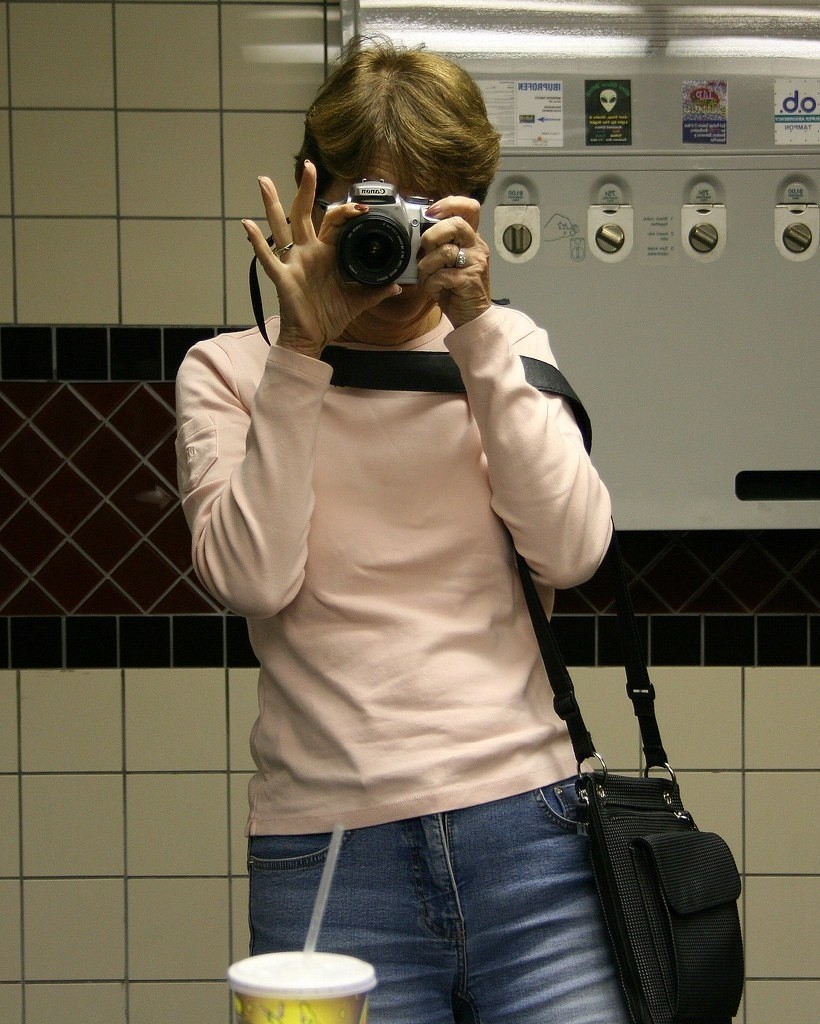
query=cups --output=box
[228,951,376,1024]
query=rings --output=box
[272,243,293,257]
[455,246,466,269]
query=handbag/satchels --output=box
[572,771,744,1024]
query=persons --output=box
[172,34,633,1024]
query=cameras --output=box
[333,178,451,286]
